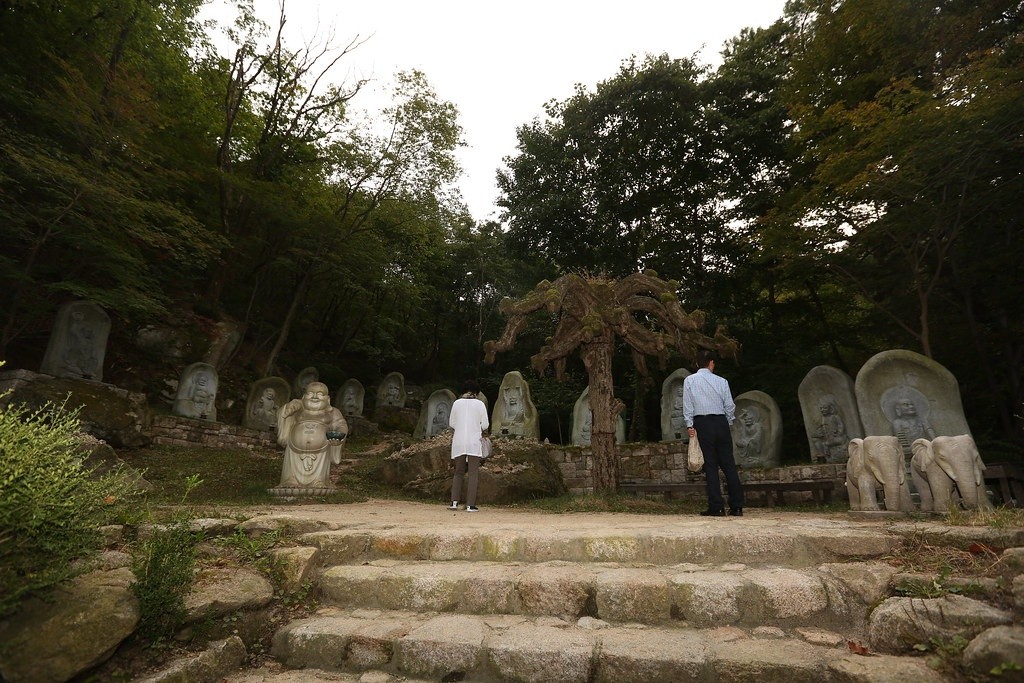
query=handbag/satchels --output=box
[688,428,704,472]
[481,430,494,458]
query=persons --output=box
[447,381,489,512]
[432,406,449,434]
[275,375,359,488]
[683,351,745,516]
[254,392,279,427]
[892,398,936,459]
[501,386,523,426]
[582,405,592,439]
[63,311,99,377]
[384,383,399,403]
[812,403,848,457]
[671,386,687,430]
[186,377,214,414]
[737,408,762,457]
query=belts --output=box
[694,414,725,418]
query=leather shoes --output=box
[700,506,725,516]
[728,507,742,515]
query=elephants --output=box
[911,434,981,512]
[846,436,914,511]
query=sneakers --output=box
[449,501,458,509]
[466,505,478,512]
